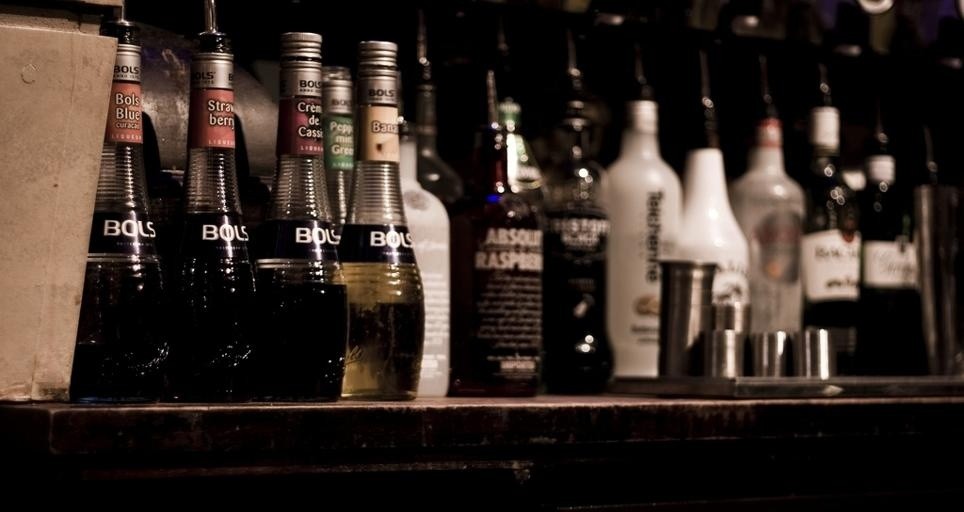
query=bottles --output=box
[170,1,268,404]
[258,31,348,404]
[71,17,184,406]
[324,4,962,393]
